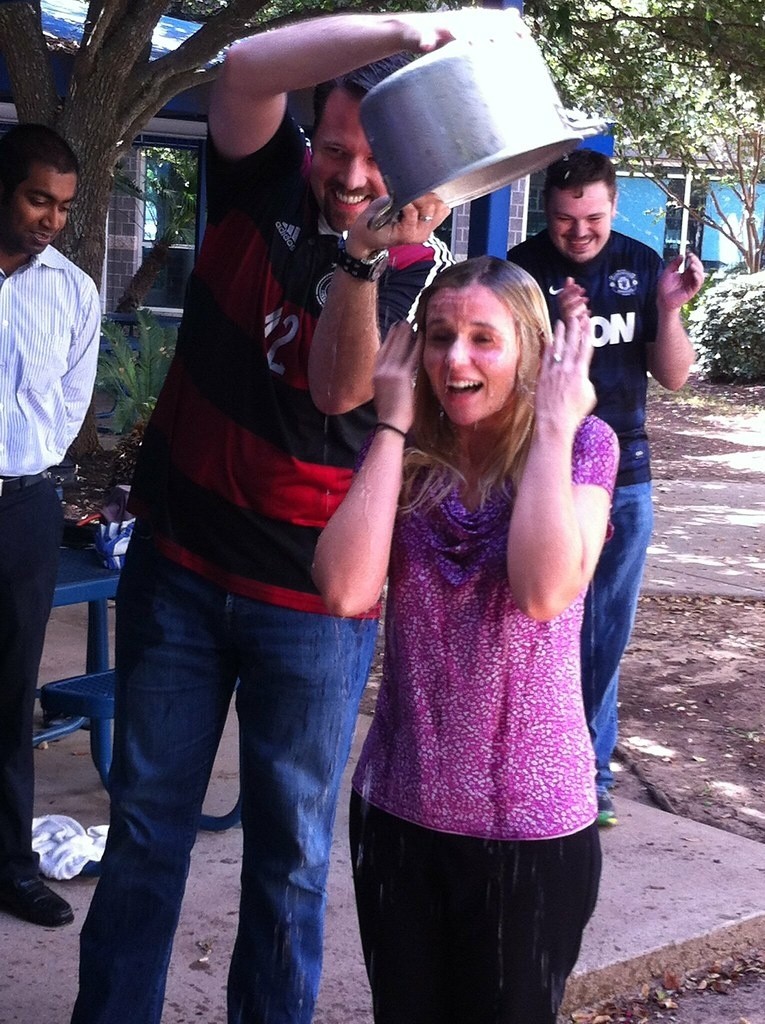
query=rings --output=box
[419,216,433,222]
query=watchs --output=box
[334,231,390,283]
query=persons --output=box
[66,8,704,1024]
[0,123,102,927]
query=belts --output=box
[0,472,47,497]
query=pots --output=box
[357,20,609,231]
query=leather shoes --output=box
[0,876,74,927]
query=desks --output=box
[52,547,121,788]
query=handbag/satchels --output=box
[94,488,137,568]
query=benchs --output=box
[41,667,240,723]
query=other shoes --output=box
[597,793,619,827]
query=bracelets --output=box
[371,419,411,443]
